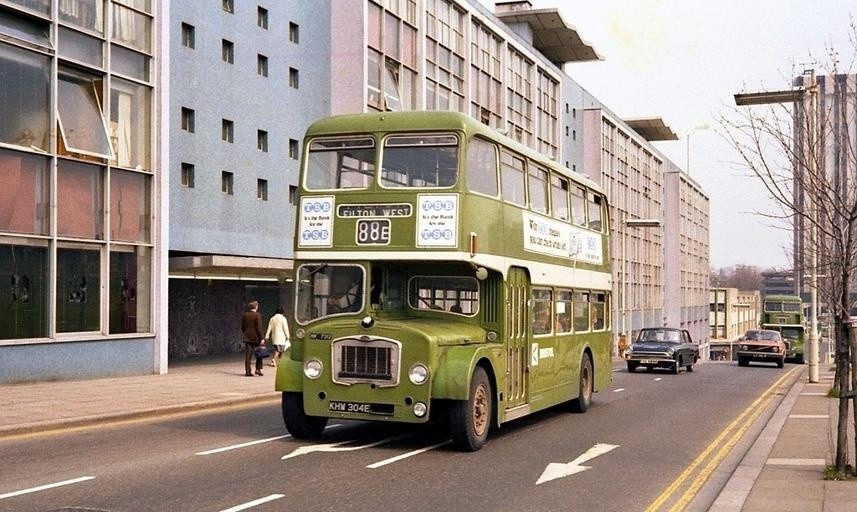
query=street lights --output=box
[792,69,820,384]
[618,218,662,360]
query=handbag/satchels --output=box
[255,345,269,358]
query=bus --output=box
[274,110,613,452]
[758,294,805,364]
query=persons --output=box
[242,301,265,377]
[265,308,290,367]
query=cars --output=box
[736,329,787,368]
[623,327,700,373]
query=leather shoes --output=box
[271,359,276,366]
[255,369,264,375]
